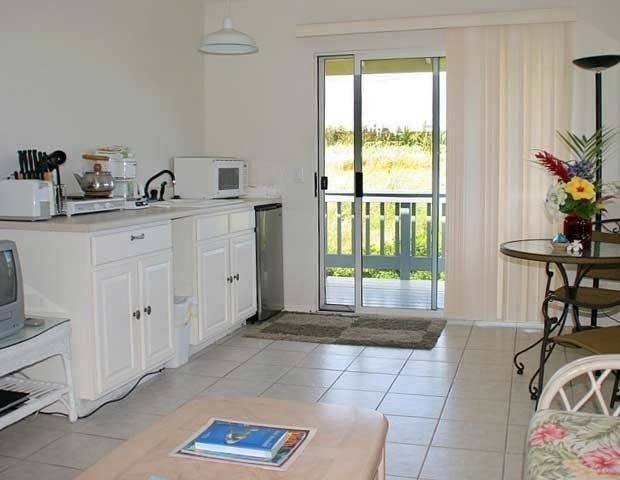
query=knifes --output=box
[15,149,50,181]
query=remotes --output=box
[24,318,44,327]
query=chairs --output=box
[535,296,620,410]
[545,218,620,328]
[523,353,620,480]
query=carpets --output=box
[240,311,447,350]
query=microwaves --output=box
[173,156,249,201]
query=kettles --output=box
[73,164,115,197]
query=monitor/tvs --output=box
[0,239,25,340]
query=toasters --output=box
[0,178,56,221]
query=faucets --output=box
[145,170,176,198]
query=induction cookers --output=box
[60,195,124,218]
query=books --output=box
[181,417,307,468]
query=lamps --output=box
[198,0,258,55]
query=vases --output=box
[564,212,592,247]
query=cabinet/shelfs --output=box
[91,219,178,397]
[192,206,258,345]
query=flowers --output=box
[528,120,620,218]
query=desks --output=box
[499,238,620,375]
[73,396,388,480]
[0,315,77,430]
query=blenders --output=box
[93,144,150,210]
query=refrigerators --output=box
[245,203,283,324]
